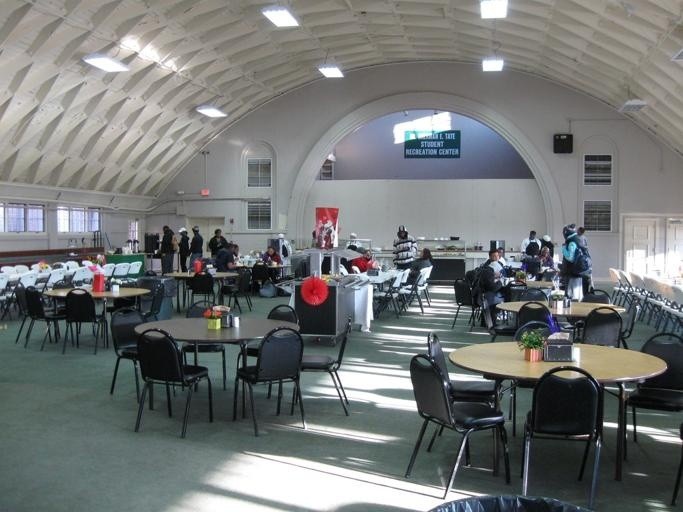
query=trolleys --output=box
[286,274,377,346]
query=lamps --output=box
[261,1,345,81]
[617,48,683,118]
[79,42,229,119]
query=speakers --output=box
[552,133,572,153]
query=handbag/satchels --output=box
[572,255,592,275]
[171,235,178,250]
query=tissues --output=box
[367,261,380,276]
[204,264,216,275]
[108,277,122,288]
[543,332,573,362]
[548,290,571,308]
[212,305,234,328]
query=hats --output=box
[563,224,576,238]
[543,235,551,241]
[278,234,285,238]
[351,233,357,239]
[178,228,186,232]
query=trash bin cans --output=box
[427,494,594,512]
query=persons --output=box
[156,225,292,283]
[476,222,593,302]
[392,223,417,269]
[398,247,436,302]
[341,245,378,274]
